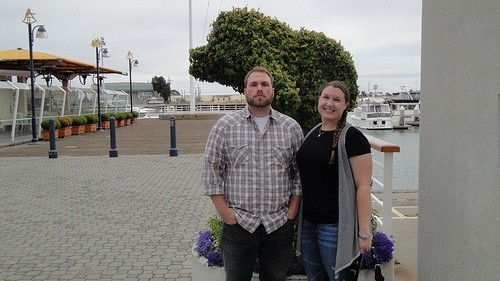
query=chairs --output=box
[0,111,39,134]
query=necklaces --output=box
[318,125,336,138]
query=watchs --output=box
[359,234,371,240]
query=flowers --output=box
[193,204,395,275]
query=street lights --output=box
[23,9,49,144]
[90,37,111,130]
[126,51,140,112]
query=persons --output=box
[295,82,372,281]
[204,67,304,281]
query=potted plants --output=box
[131,111,139,124]
[85,114,99,133]
[72,115,87,136]
[116,113,127,127]
[124,112,134,126]
[58,117,74,138]
[108,112,118,128]
[40,119,61,141]
[100,114,110,130]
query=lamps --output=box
[79,74,88,85]
[41,74,53,87]
[92,74,107,86]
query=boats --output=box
[347,99,393,130]
